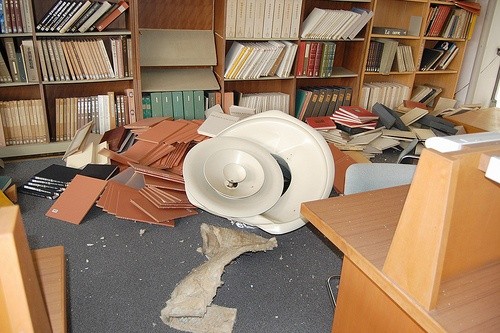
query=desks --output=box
[1,204,68,333]
[300,131,500,333]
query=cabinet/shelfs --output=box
[0,0,481,165]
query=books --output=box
[0,0,480,228]
[45,174,108,225]
